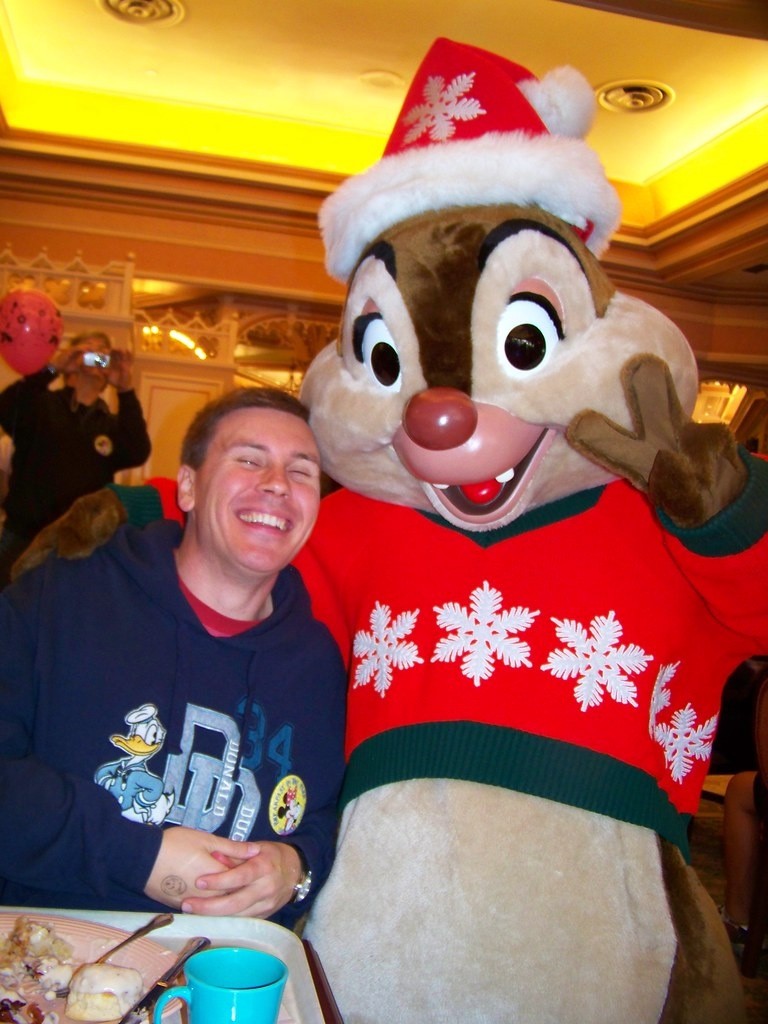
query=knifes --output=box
[117,937,210,1024]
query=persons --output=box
[0,387,347,933]
[716,769,768,962]
[0,332,152,573]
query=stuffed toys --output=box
[9,38,768,1024]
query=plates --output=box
[0,912,186,1024]
[0,905,325,1024]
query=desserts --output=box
[66,962,144,1023]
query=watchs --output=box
[287,843,312,904]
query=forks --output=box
[37,912,174,998]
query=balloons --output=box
[0,288,64,377]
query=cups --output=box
[153,947,289,1024]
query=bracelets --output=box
[47,363,58,375]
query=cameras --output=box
[84,352,110,368]
